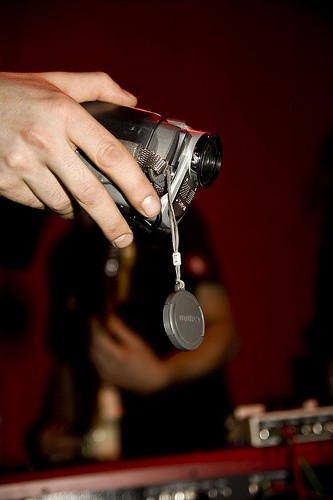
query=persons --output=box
[1,70,161,248]
[225,145,333,444]
[23,198,235,466]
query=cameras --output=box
[74,99,225,232]
